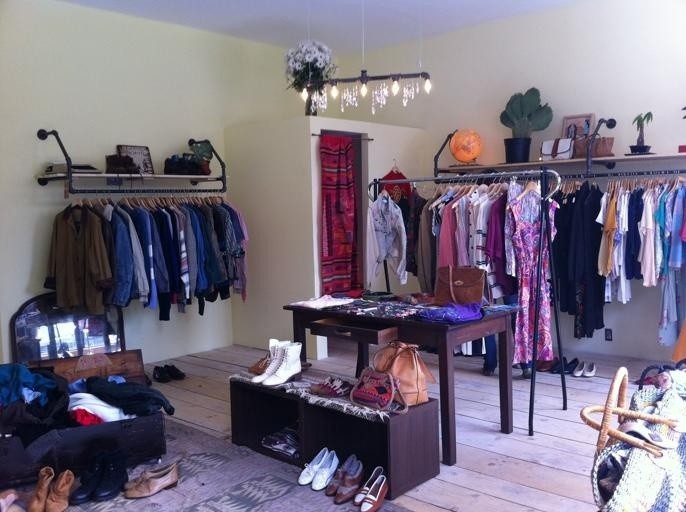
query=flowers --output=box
[280,39,337,77]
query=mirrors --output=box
[8,287,125,365]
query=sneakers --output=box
[104,154,141,175]
[164,154,207,175]
[152,364,187,383]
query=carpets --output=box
[0,410,410,511]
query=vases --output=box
[296,67,323,86]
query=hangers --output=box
[52,185,237,218]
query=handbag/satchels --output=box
[539,137,576,162]
[349,339,439,417]
[579,357,686,512]
[432,264,494,309]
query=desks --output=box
[283,291,517,467]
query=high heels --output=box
[481,367,495,377]
[523,367,532,379]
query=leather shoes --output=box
[296,446,391,512]
[536,355,598,378]
[122,461,180,500]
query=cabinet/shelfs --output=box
[229,368,442,499]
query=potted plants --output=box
[628,112,655,154]
[500,82,557,166]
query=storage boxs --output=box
[50,411,167,483]
[0,434,31,482]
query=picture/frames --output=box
[562,114,594,139]
[115,142,156,175]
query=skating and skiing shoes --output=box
[309,376,355,399]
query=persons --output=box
[582,119,589,139]
[568,119,577,138]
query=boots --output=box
[26,443,129,512]
[247,337,313,388]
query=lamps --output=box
[300,1,441,115]
